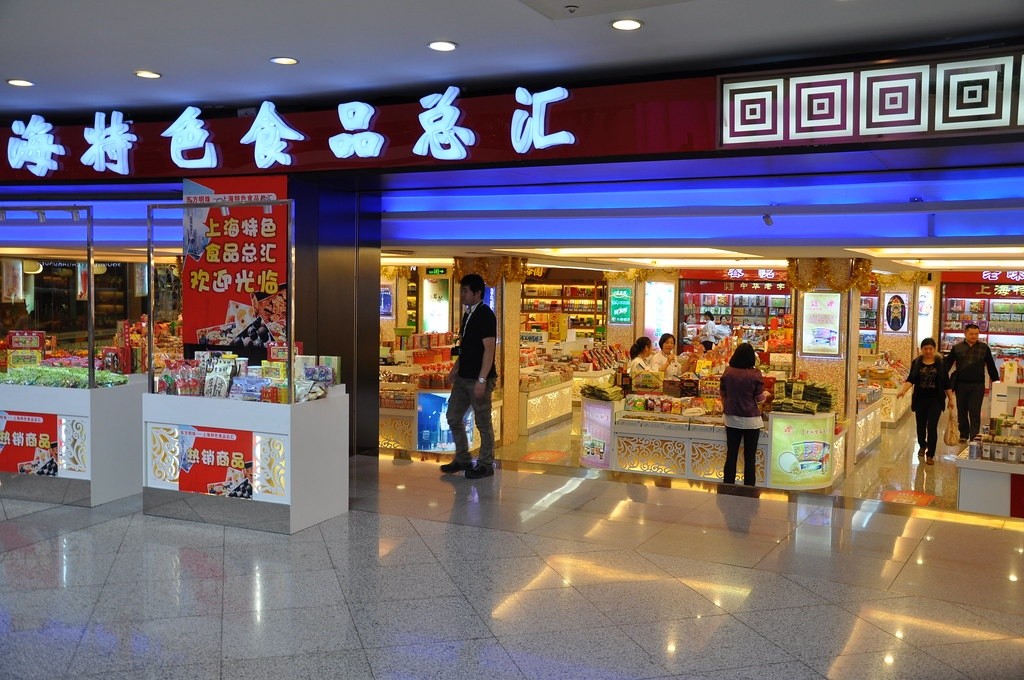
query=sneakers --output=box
[465,460,494,479]
[441,461,473,473]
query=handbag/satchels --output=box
[944,407,961,446]
[950,371,958,390]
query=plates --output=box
[779,452,798,473]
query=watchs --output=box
[476,378,487,384]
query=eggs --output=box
[235,306,251,328]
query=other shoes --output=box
[927,457,934,464]
[918,442,928,456]
[960,438,967,442]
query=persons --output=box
[15,310,35,329]
[720,343,767,486]
[651,333,696,376]
[945,324,1001,442]
[630,337,652,371]
[700,311,716,350]
[897,338,954,464]
[716,315,731,344]
[440,274,498,480]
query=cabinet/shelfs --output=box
[937,281,1024,357]
[521,268,607,343]
[684,280,792,328]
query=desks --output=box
[0,373,150,508]
[142,384,349,535]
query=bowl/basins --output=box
[394,327,413,336]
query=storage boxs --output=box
[0,331,56,369]
[261,342,341,386]
[101,320,147,374]
